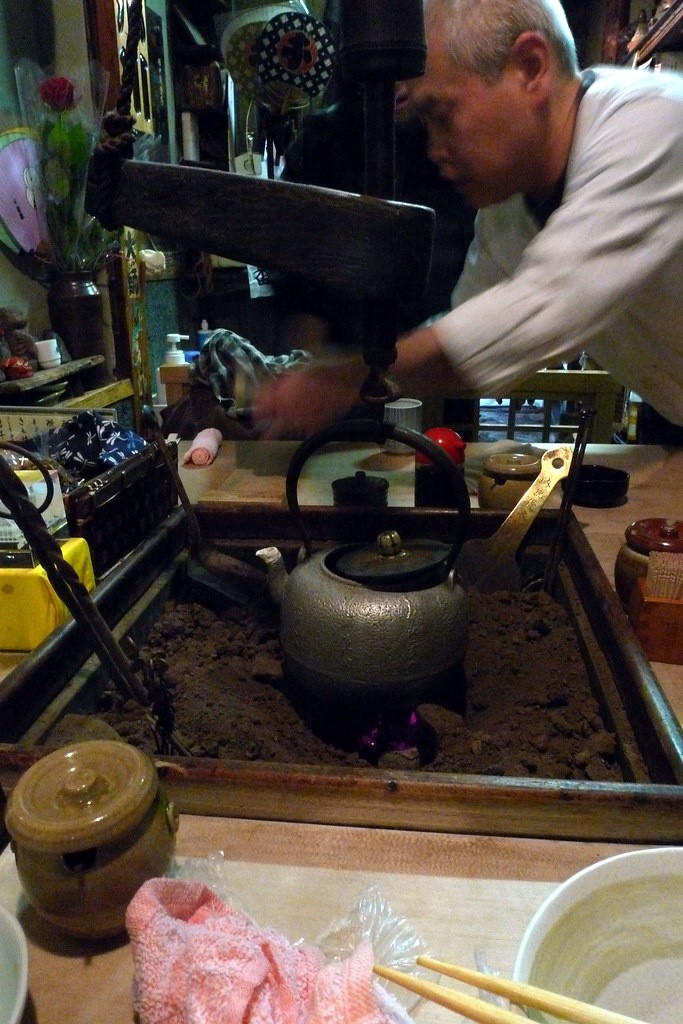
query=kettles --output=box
[255,420,475,699]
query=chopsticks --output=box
[370,955,652,1024]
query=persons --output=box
[251,0,683,443]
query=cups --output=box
[330,471,390,507]
[34,338,61,369]
[384,396,422,455]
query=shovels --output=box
[141,403,269,605]
[450,452,571,595]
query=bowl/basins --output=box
[511,848,683,1024]
[562,463,630,505]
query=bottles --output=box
[196,317,213,347]
[412,426,470,506]
